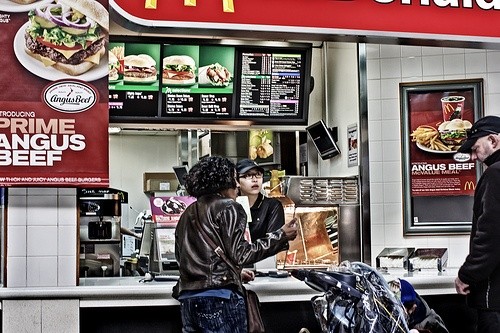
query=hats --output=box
[457,115,500,153]
[235,158,265,174]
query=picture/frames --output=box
[400,78,484,236]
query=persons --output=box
[234,158,290,277]
[454,116,500,333]
[172,154,298,333]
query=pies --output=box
[199,62,230,87]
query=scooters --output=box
[289,260,449,333]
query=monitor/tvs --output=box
[306,120,341,160]
[172,165,190,189]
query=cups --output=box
[441,96,465,121]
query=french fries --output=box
[411,127,452,151]
[110,46,124,60]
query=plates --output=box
[13,20,109,81]
[416,140,458,153]
[0,0,54,12]
[160,201,187,216]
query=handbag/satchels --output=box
[245,289,266,333]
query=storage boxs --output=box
[144,171,177,192]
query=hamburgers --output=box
[109,51,120,80]
[162,55,196,85]
[24,0,109,77]
[438,119,473,143]
[123,54,156,83]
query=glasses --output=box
[238,171,264,179]
